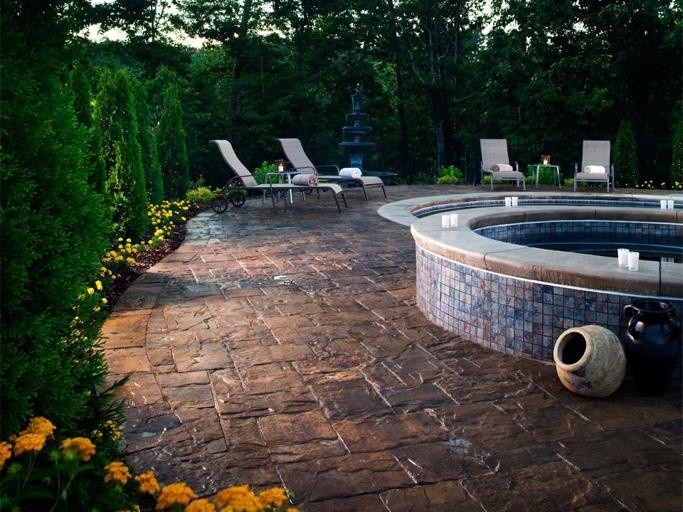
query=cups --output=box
[660,200,673,209]
[504,196,517,206]
[616,247,638,272]
[440,213,458,229]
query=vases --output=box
[553,325,625,398]
[621,298,682,397]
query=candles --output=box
[543,158,546,164]
[278,164,283,172]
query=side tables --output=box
[263,170,305,205]
[530,164,561,190]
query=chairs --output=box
[573,140,614,192]
[208,138,347,214]
[276,137,388,200]
[479,138,526,191]
